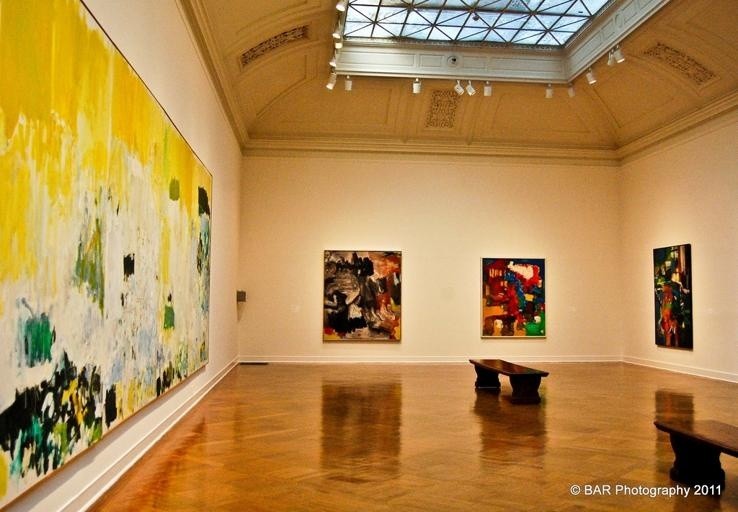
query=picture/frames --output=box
[319,247,403,348]
[476,255,550,344]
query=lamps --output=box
[325,1,629,104]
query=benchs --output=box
[651,417,738,491]
[466,357,551,404]
